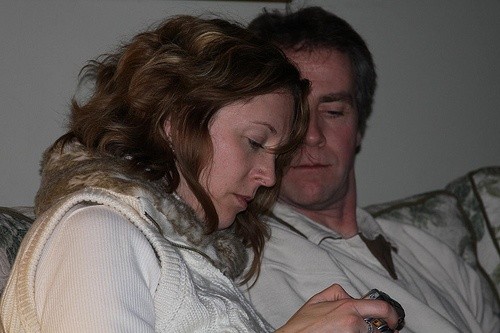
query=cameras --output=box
[360,288,405,333]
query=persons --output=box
[0,15,402,333]
[240,6,500,333]
